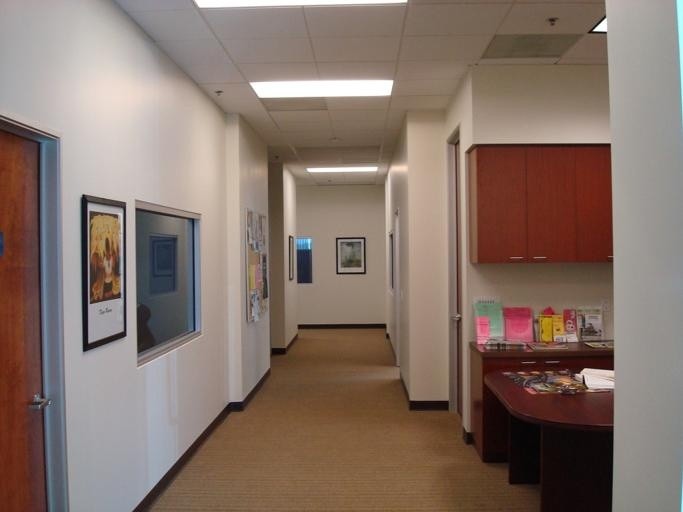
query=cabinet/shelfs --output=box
[468,342,614,462]
[472,147,614,264]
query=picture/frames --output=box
[336,237,366,274]
[82,194,127,353]
[289,236,295,280]
[152,240,175,277]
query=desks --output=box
[484,371,613,512]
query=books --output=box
[574,367,615,389]
